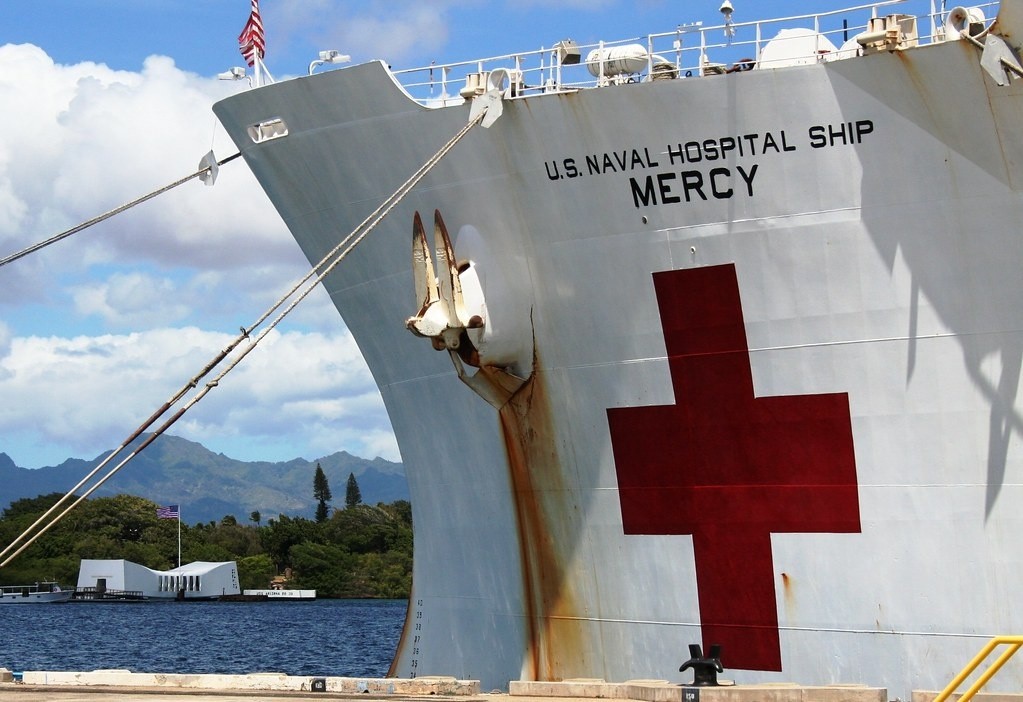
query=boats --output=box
[0,580,74,604]
[212,0,1023,702]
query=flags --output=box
[156,505,180,519]
[237,0,266,67]
